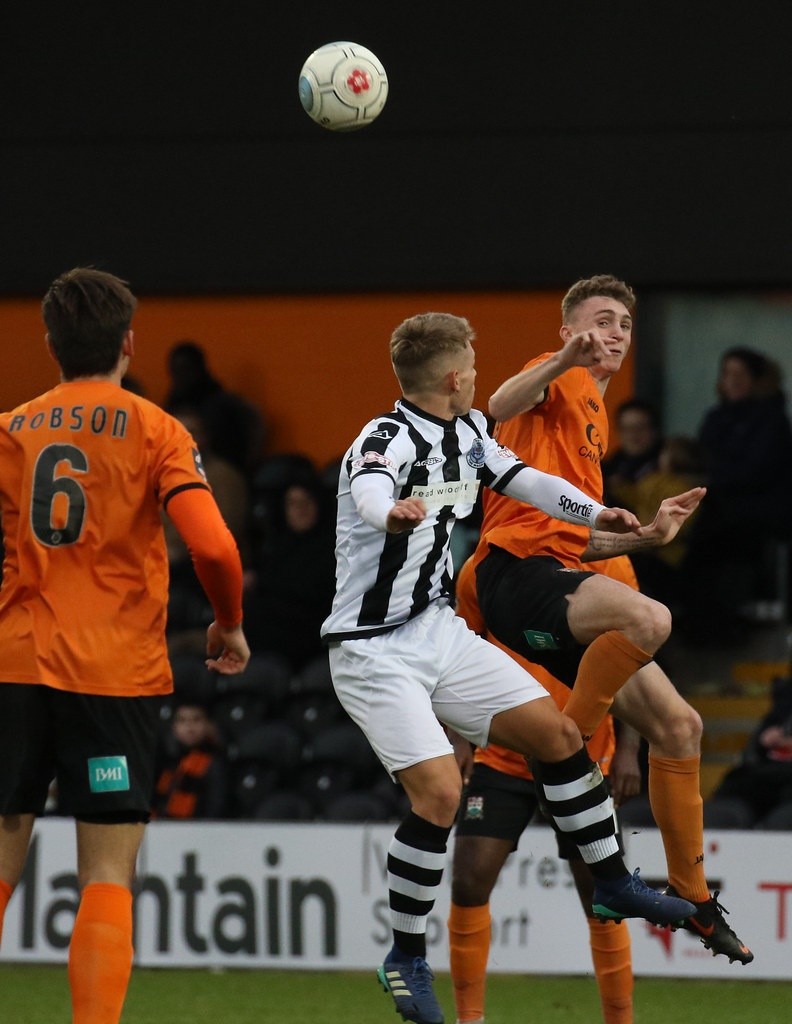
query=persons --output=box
[319,312,708,1024]
[0,267,251,1024]
[447,555,641,1024]
[470,276,754,966]
[147,341,413,820]
[605,347,792,829]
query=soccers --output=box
[298,40,391,132]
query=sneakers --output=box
[523,756,562,835]
[662,881,754,966]
[377,950,445,1024]
[592,866,697,927]
